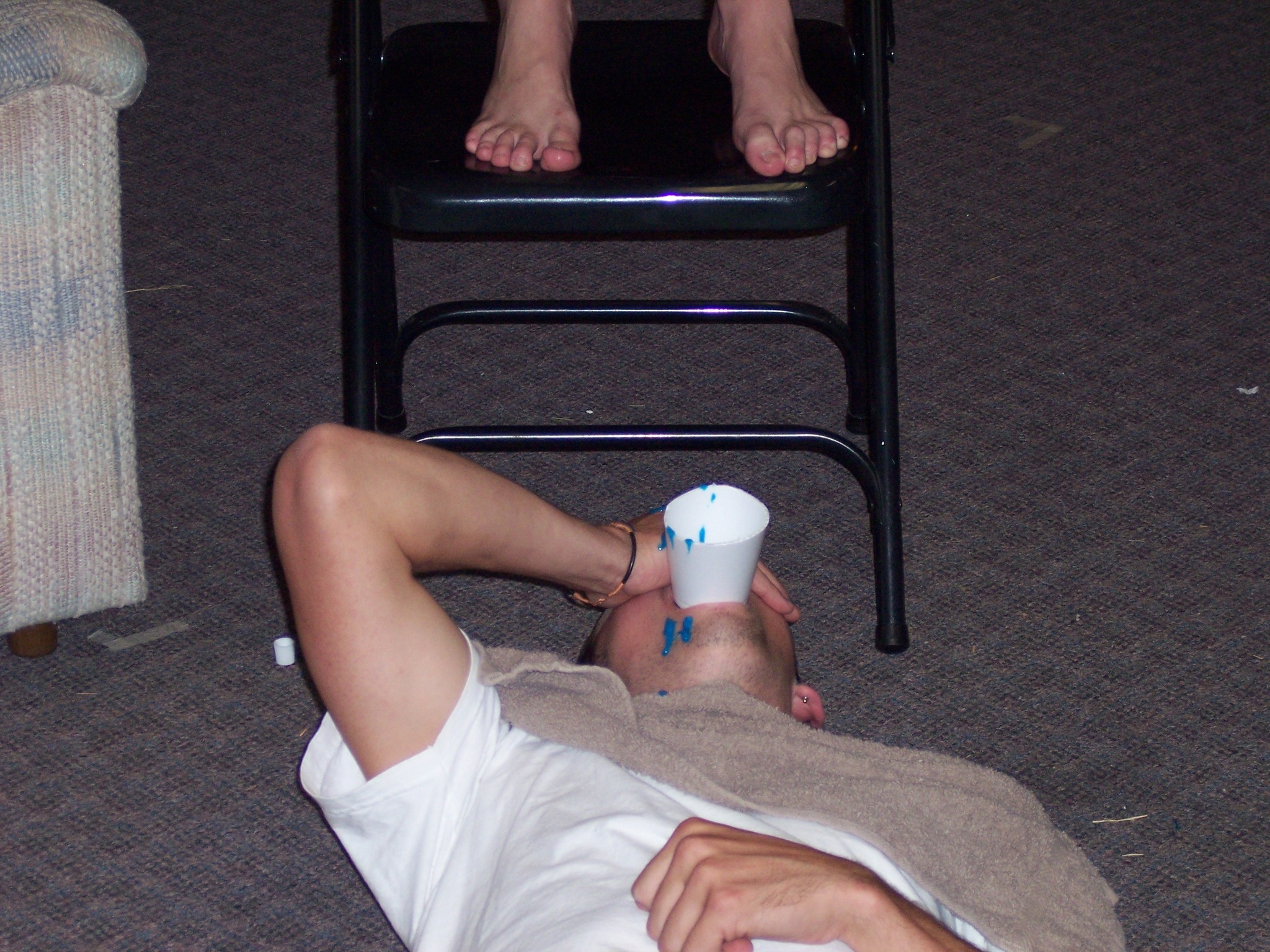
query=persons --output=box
[270,423,1127,952]
[465,0,849,178]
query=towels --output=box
[470,634,1126,951]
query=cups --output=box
[664,485,770,608]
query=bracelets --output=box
[571,521,637,606]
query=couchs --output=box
[0,0,149,657]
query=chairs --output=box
[328,0,910,654]
